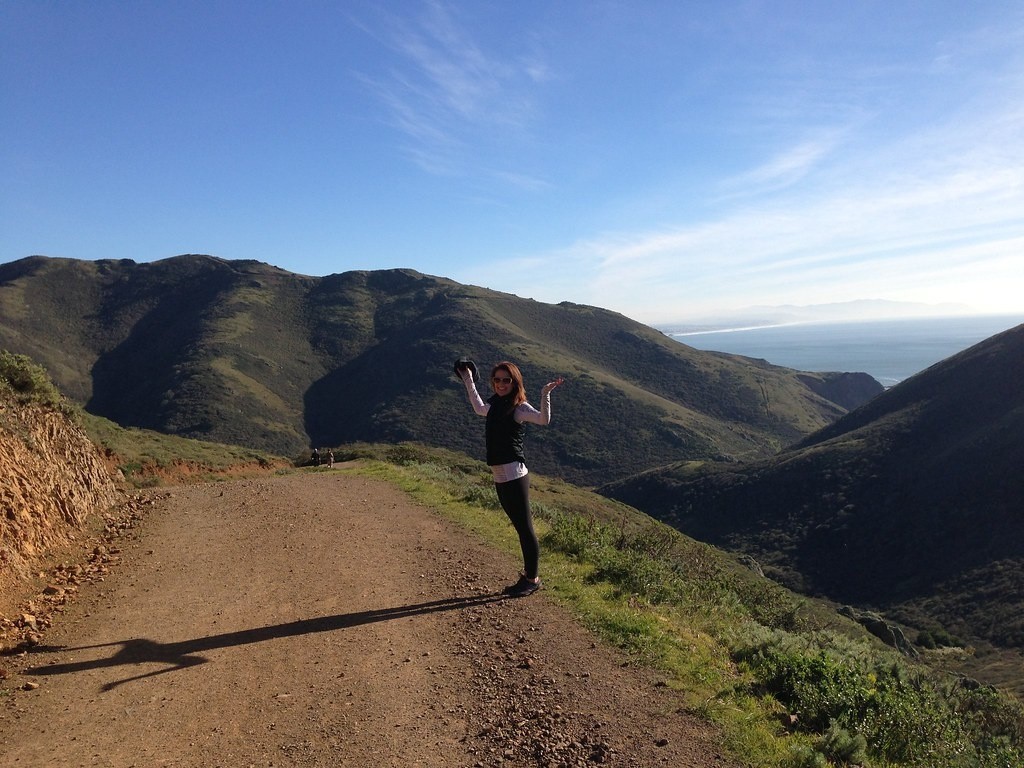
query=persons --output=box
[458,361,563,597]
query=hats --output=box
[454,359,480,382]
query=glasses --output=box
[492,377,513,384]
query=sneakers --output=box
[506,575,542,598]
